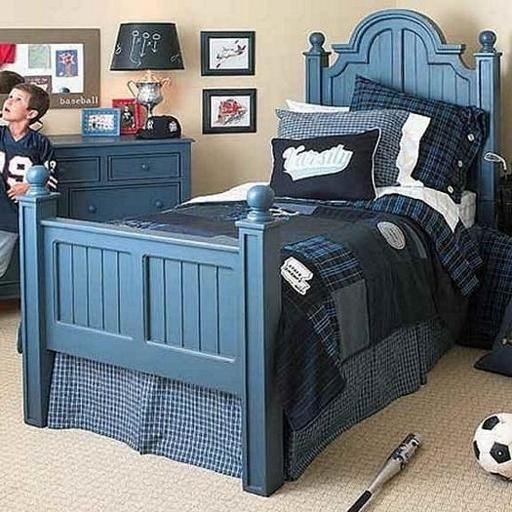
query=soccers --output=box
[470,411,512,482]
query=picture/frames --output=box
[202,88,257,135]
[0,26,100,111]
[200,30,254,77]
[81,110,120,136]
[111,97,142,134]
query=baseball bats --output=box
[346,432,422,512]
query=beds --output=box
[16,10,501,498]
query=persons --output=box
[0,83,58,278]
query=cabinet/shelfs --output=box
[0,134,195,303]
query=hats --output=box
[138,117,181,139]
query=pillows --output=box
[287,99,430,188]
[275,107,409,187]
[475,289,512,379]
[268,128,382,201]
[471,224,511,347]
[347,76,490,200]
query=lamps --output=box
[110,22,184,134]
